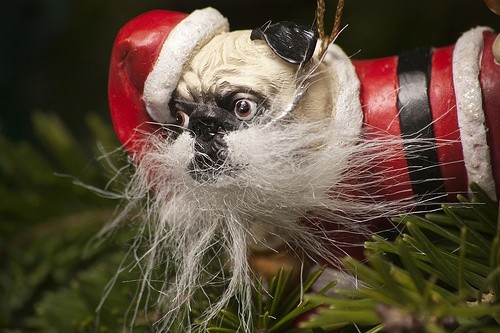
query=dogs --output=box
[168,20,500,333]
